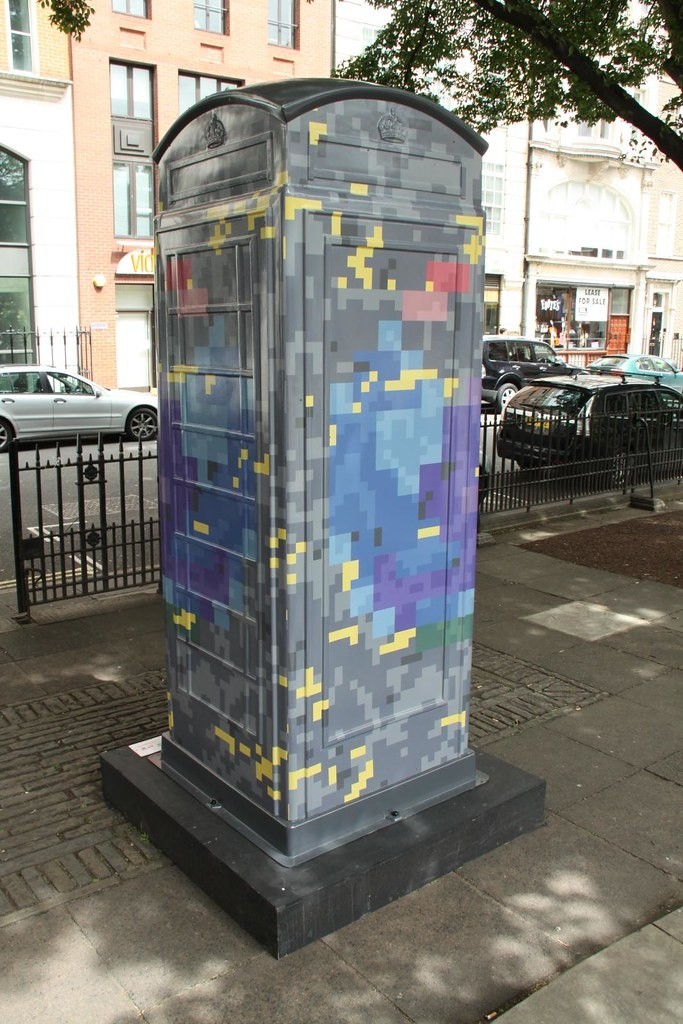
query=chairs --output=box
[13,377,29,393]
[638,364,650,370]
[32,378,44,393]
[511,347,531,362]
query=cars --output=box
[584,353,683,402]
[0,364,158,454]
[480,335,593,413]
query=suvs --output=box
[497,369,683,487]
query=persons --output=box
[500,328,507,334]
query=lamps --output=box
[91,273,106,287]
